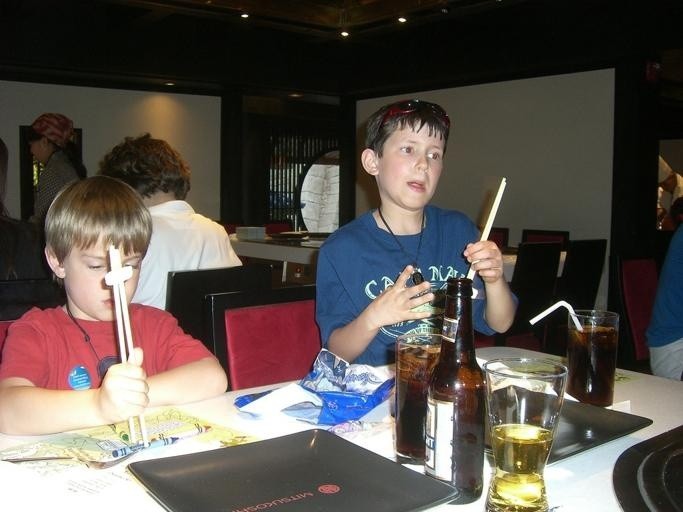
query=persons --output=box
[95,132,244,313]
[648,221,682,381]
[316,98,520,369]
[0,140,55,280]
[25,113,85,226]
[670,195,683,225]
[0,173,228,437]
[656,155,683,232]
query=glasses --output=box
[368,98,451,149]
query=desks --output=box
[230,236,328,285]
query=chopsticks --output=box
[467,176,508,282]
[105,244,154,447]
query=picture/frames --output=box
[18,123,83,219]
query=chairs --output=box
[264,223,290,235]
[165,263,272,346]
[223,224,248,265]
[475,226,660,374]
[0,279,67,321]
[0,320,14,355]
[204,283,322,392]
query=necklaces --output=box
[64,304,121,380]
[376,206,426,286]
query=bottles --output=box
[422,275,487,505]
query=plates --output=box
[127,428,460,511]
[484,384,654,466]
[235,226,267,241]
[268,231,310,241]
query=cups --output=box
[394,325,443,464]
[564,307,621,408]
[482,356,573,512]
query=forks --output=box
[0,445,143,472]
